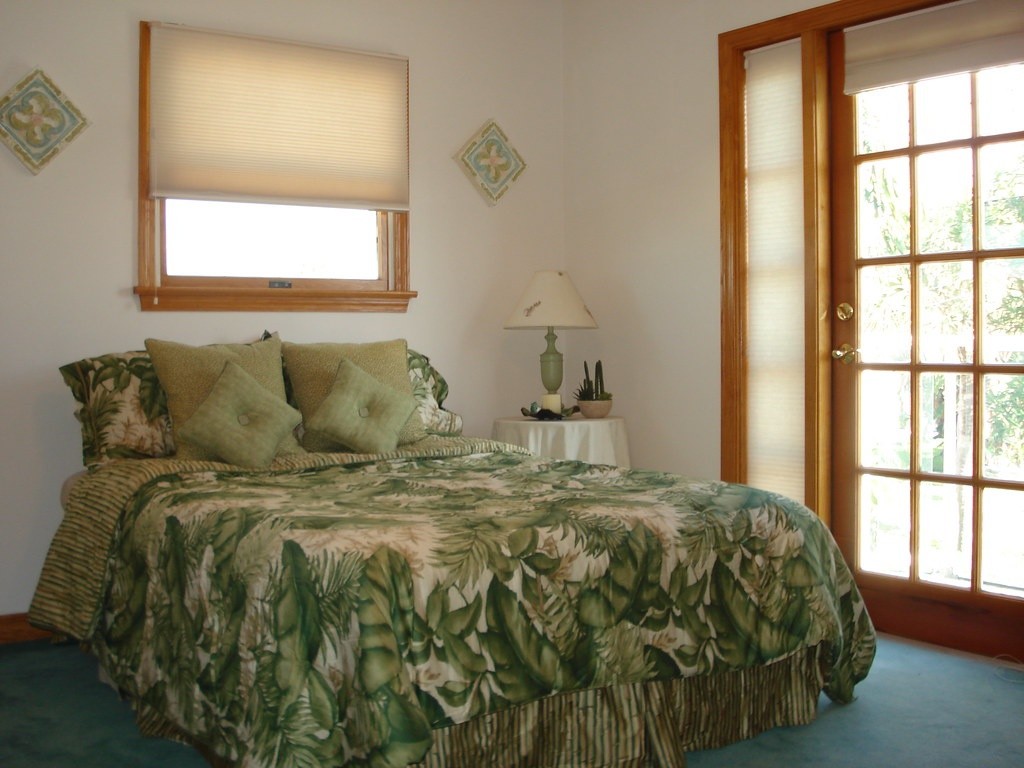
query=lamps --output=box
[501,269,598,415]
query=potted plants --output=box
[571,359,614,419]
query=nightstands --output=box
[493,419,631,471]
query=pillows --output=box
[280,340,429,454]
[304,355,419,455]
[407,348,463,439]
[175,359,302,471]
[57,351,175,470]
[145,330,304,461]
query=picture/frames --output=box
[0,69,91,179]
[455,118,528,207]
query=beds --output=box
[25,436,878,768]
